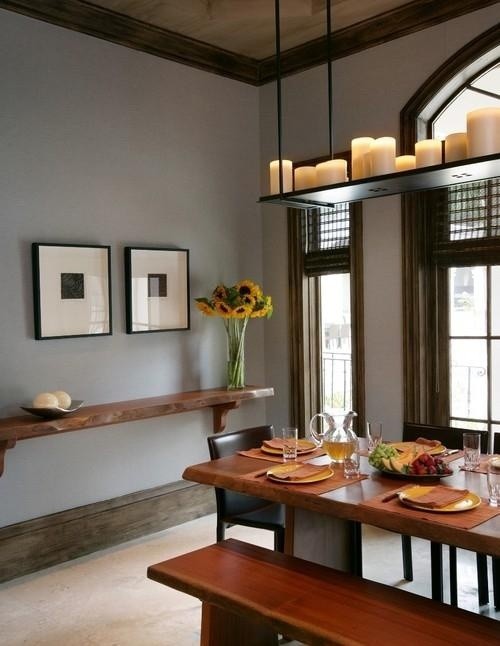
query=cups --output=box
[487,461,500,506]
[282,427,297,461]
[367,421,383,453]
[343,440,360,478]
[462,432,481,472]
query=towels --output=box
[404,485,470,508]
[263,438,313,452]
[270,464,330,480]
[396,438,441,453]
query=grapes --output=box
[368,443,399,470]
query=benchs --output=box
[148,539,500,645]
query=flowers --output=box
[193,279,274,320]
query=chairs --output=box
[401,423,489,605]
[207,424,287,555]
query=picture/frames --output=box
[32,241,114,341]
[123,246,192,335]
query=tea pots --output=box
[310,410,359,461]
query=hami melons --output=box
[382,445,425,472]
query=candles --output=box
[373,137,396,177]
[350,137,373,181]
[317,159,347,187]
[415,139,442,169]
[467,108,500,159]
[396,155,416,172]
[269,160,292,196]
[294,166,317,191]
[444,133,466,163]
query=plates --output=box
[18,400,86,418]
[387,441,447,456]
[261,438,317,456]
[372,454,454,478]
[266,463,334,485]
[399,486,482,513]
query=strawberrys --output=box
[405,453,451,477]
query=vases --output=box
[223,317,248,392]
